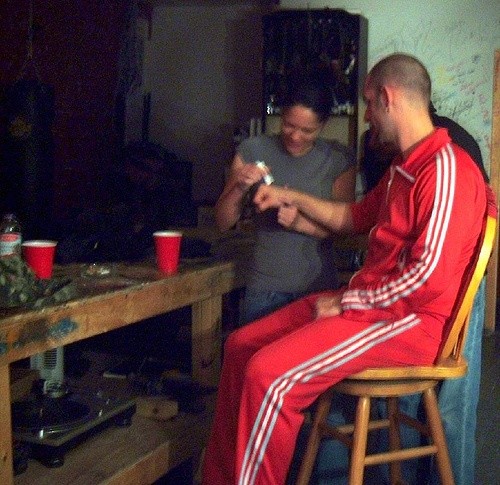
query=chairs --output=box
[293,216,497,485]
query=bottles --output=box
[0,214,22,261]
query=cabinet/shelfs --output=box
[261,8,367,164]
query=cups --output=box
[153,232,182,274]
[21,240,56,280]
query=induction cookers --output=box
[9,374,137,469]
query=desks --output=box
[0,226,258,484]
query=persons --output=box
[360,100,490,485]
[213,84,357,484]
[200,53,488,484]
